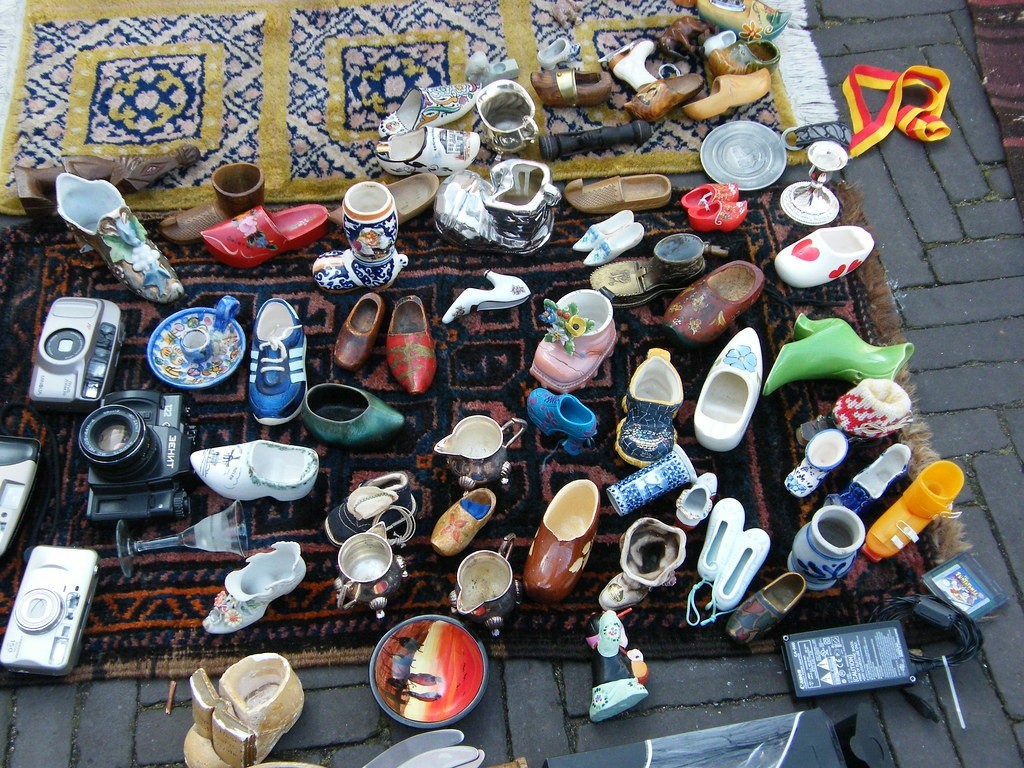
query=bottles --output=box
[787,504,866,591]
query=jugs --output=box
[475,78,539,154]
[456,532,518,625]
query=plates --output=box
[700,120,787,191]
[146,292,246,390]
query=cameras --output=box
[0,432,43,556]
[29,296,126,414]
[78,389,192,524]
[0,543,101,677]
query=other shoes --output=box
[0,0,965,768]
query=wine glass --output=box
[116,500,250,577]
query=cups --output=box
[606,444,698,518]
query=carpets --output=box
[0,1,1024,682]
